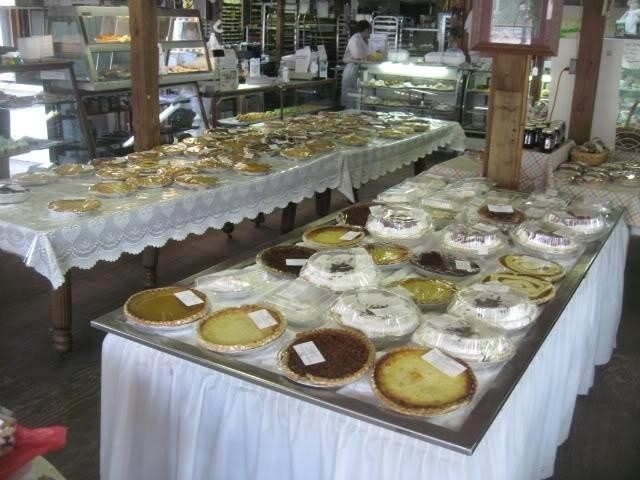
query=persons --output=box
[340,20,377,110]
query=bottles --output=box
[278,60,290,84]
[523,119,566,154]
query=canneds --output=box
[522,118,566,153]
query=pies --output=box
[124,172,608,420]
[1,110,431,214]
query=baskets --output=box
[570,146,609,166]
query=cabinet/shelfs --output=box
[41,5,214,90]
[1,58,99,176]
[44,80,210,163]
